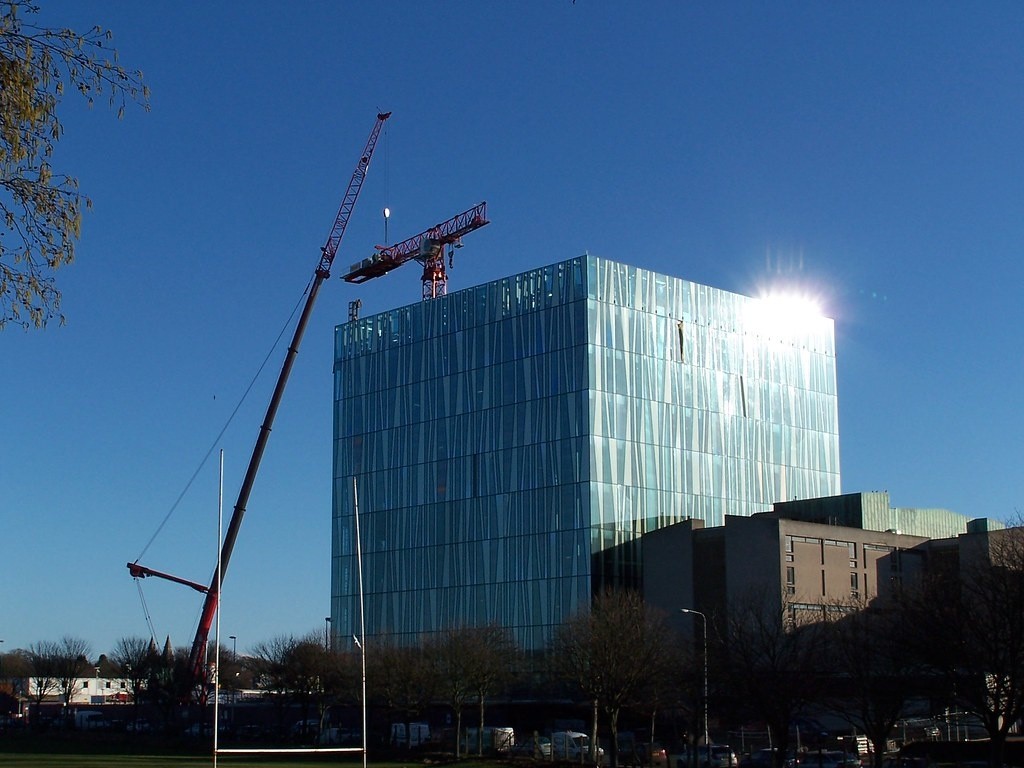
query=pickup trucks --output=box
[319,728,356,745]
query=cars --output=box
[739,747,798,768]
[523,737,551,756]
[617,742,667,768]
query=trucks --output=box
[459,727,515,755]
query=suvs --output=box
[677,745,737,768]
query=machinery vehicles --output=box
[127,107,395,731]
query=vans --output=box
[793,751,863,768]
[75,710,108,728]
[553,731,604,760]
[391,722,430,748]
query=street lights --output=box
[229,636,236,700]
[94,667,100,695]
[325,617,331,652]
[680,608,708,745]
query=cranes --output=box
[339,201,491,300]
